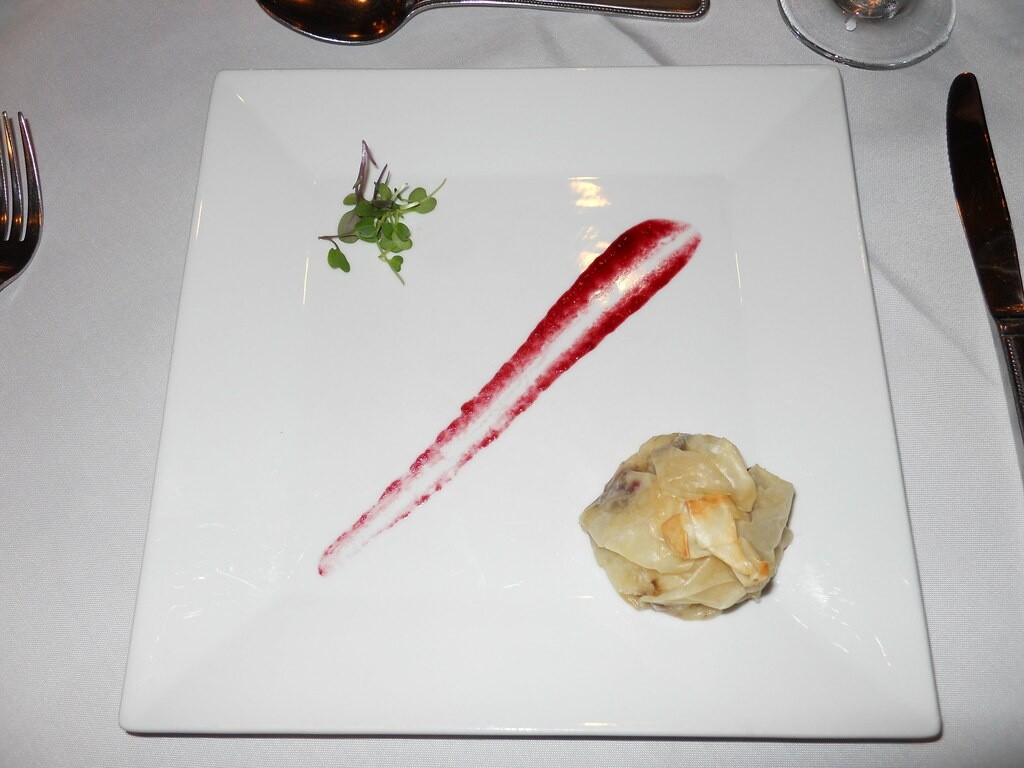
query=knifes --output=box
[946,72,1024,448]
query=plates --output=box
[120,64,943,739]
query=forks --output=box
[0,111,44,292]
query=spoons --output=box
[255,0,712,46]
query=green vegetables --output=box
[318,139,447,285]
